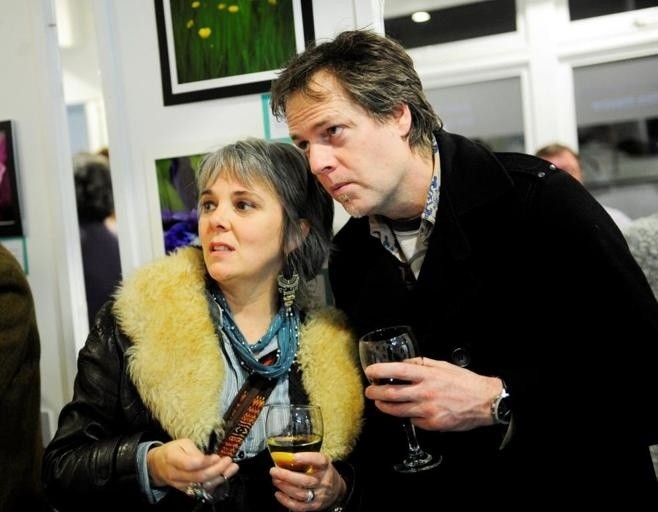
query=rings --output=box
[219,474,228,479]
[303,487,315,504]
[185,480,214,502]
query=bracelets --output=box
[318,471,351,511]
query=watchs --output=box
[487,375,511,429]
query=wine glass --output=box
[261,403,325,511]
[358,324,443,474]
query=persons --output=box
[69,158,122,323]
[40,136,368,512]
[0,126,45,512]
[530,143,634,233]
[268,28,657,512]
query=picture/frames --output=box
[151,0,319,110]
